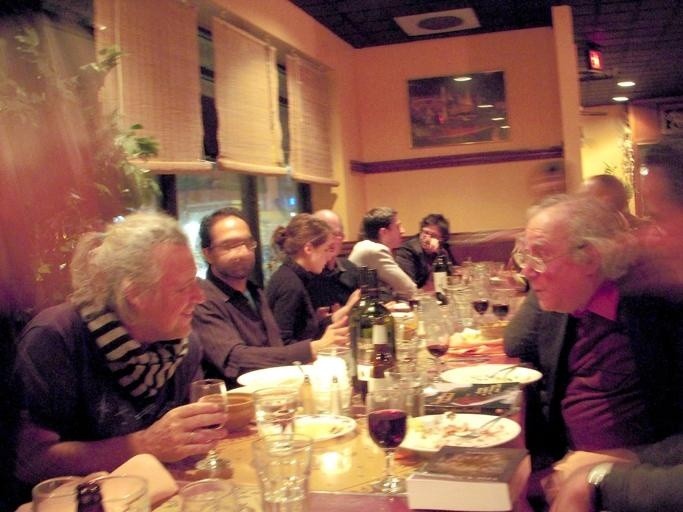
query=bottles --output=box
[432,258,449,302]
[356,269,397,405]
[330,376,345,414]
[346,265,371,396]
[76,482,107,512]
[297,376,317,416]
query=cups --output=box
[182,478,253,512]
[253,434,311,510]
[254,387,296,435]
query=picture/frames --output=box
[658,102,682,137]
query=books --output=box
[408,444,531,511]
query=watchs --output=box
[585,462,615,512]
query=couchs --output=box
[445,230,526,272]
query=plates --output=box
[265,413,358,444]
[387,410,524,455]
[439,362,543,389]
[235,364,351,391]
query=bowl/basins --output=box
[195,392,254,435]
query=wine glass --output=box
[425,320,452,380]
[191,379,226,476]
[470,290,489,321]
[33,475,83,512]
[366,389,407,494]
[491,291,511,324]
[91,474,151,510]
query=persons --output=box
[262,214,344,348]
[539,430,683,512]
[503,143,683,364]
[514,193,683,477]
[395,213,459,288]
[187,207,350,393]
[3,212,228,483]
[348,205,418,298]
[312,209,390,340]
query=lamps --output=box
[583,40,603,72]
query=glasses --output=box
[206,238,257,252]
[335,232,343,242]
[514,244,596,274]
